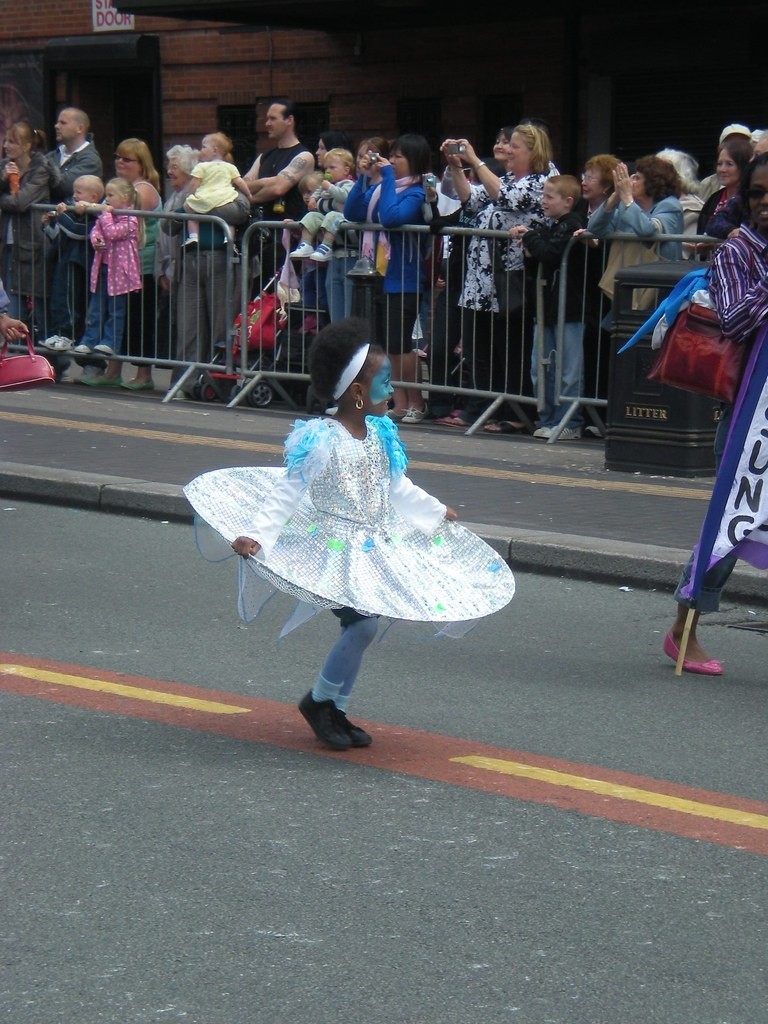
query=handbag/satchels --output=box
[646,236,754,403]
[598,218,669,310]
[493,268,529,314]
[0,329,56,392]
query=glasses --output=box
[113,153,137,162]
[748,190,766,198]
[581,173,598,182]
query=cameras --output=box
[368,153,379,162]
[423,175,437,187]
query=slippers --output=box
[485,422,536,435]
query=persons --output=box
[230,320,459,751]
[0,100,768,443]
[659,152,768,675]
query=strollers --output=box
[190,243,304,407]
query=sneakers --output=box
[299,689,353,747]
[385,410,406,421]
[534,426,581,439]
[336,709,372,745]
[289,242,314,260]
[402,403,429,423]
[310,244,333,262]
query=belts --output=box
[332,250,360,259]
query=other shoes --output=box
[586,426,602,437]
[433,410,472,428]
[119,379,155,391]
[75,345,92,353]
[325,407,338,415]
[74,365,104,383]
[180,237,198,252]
[80,377,123,387]
[663,633,722,674]
[224,238,240,256]
[39,335,73,350]
[94,345,113,354]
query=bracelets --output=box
[475,162,486,173]
[92,202,97,207]
[0,312,6,319]
[625,200,634,207]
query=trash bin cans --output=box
[605,258,724,480]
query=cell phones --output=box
[446,142,460,155]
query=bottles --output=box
[9,172,20,195]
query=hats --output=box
[720,125,752,144]
[751,130,764,142]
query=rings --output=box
[618,177,623,181]
[80,204,83,207]
